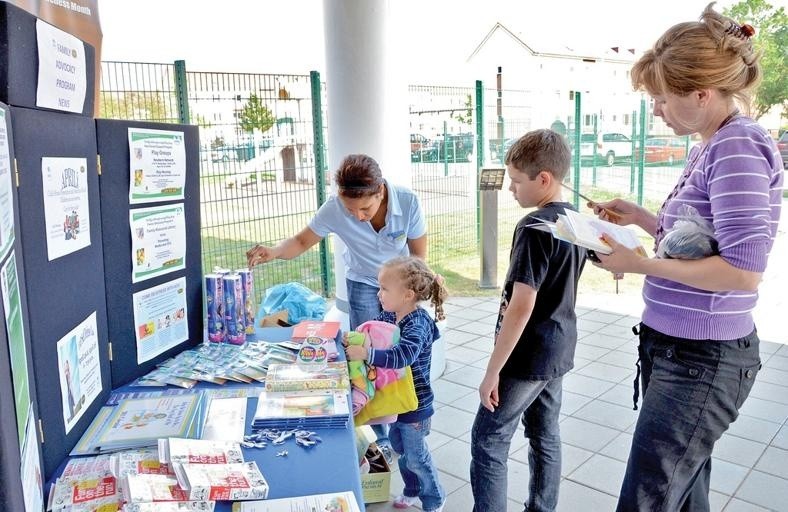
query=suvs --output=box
[464,135,498,163]
[569,132,640,167]
[776,131,787,168]
[410,134,431,156]
[447,135,468,153]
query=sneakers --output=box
[393,493,419,507]
[424,496,446,512]
[380,445,393,464]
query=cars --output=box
[496,139,517,163]
[636,137,686,165]
[211,146,238,162]
[415,140,460,162]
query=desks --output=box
[46,327,366,512]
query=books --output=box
[525,206,649,260]
[68,392,207,455]
[266,361,350,396]
[232,490,360,512]
[47,438,270,511]
[296,337,339,365]
[251,389,350,430]
[129,342,302,388]
[293,321,341,342]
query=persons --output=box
[342,256,447,512]
[247,155,427,467]
[470,129,623,512]
[587,1,784,512]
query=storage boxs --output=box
[356,425,393,503]
